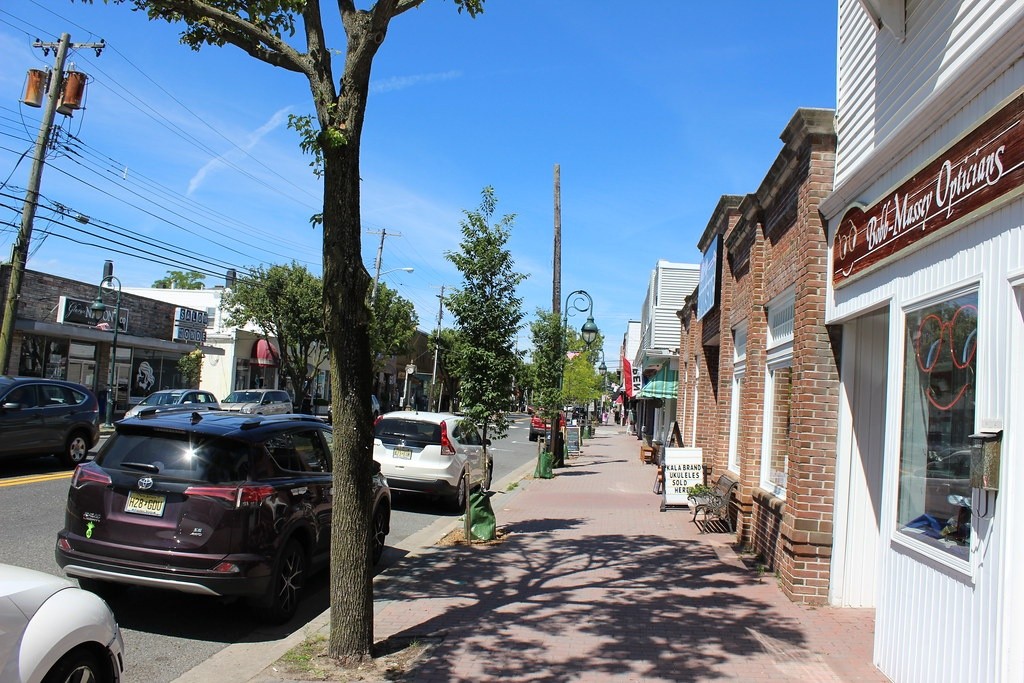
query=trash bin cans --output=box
[581,426,591,439]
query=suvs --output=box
[528,410,570,442]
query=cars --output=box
[328,395,381,425]
[372,410,494,513]
[572,407,588,419]
[123,389,223,420]
[219,389,294,418]
[564,404,574,412]
[54,404,391,631]
[0,561,127,683]
[0,376,100,465]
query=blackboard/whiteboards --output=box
[563,427,580,452]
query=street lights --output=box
[589,347,607,376]
[557,289,599,413]
[89,275,122,429]
[370,268,415,310]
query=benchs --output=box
[687,471,739,534]
[640,446,655,462]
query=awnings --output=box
[635,362,678,398]
[249,338,280,368]
